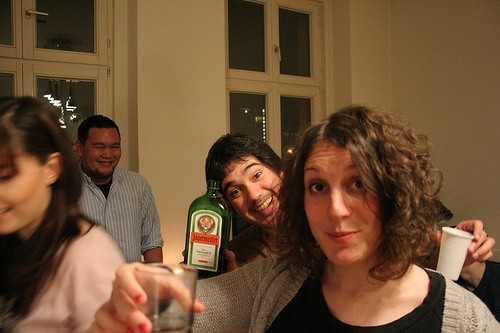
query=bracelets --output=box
[159,264,185,274]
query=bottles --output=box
[184,180,231,277]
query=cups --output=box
[436,227,474,281]
[134,262,197,333]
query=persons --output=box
[76,115,164,263]
[1,96,127,333]
[88,104,500,333]
